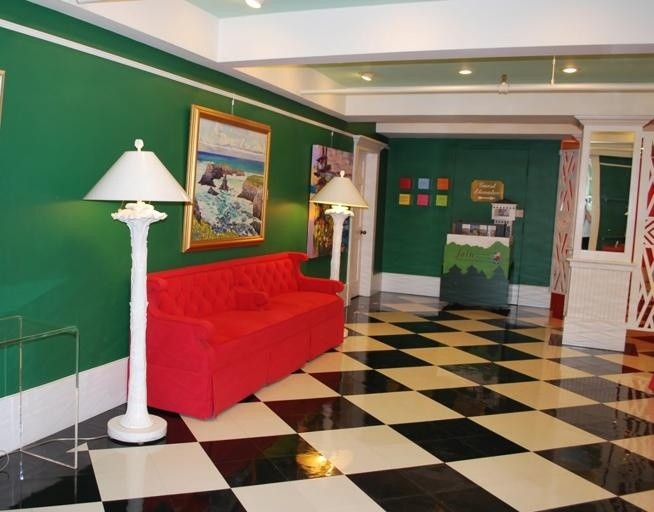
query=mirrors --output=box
[581,130,634,251]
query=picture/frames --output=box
[180,103,271,254]
[305,144,353,260]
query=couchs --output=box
[125,251,345,421]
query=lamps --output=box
[307,169,369,338]
[81,137,192,447]
